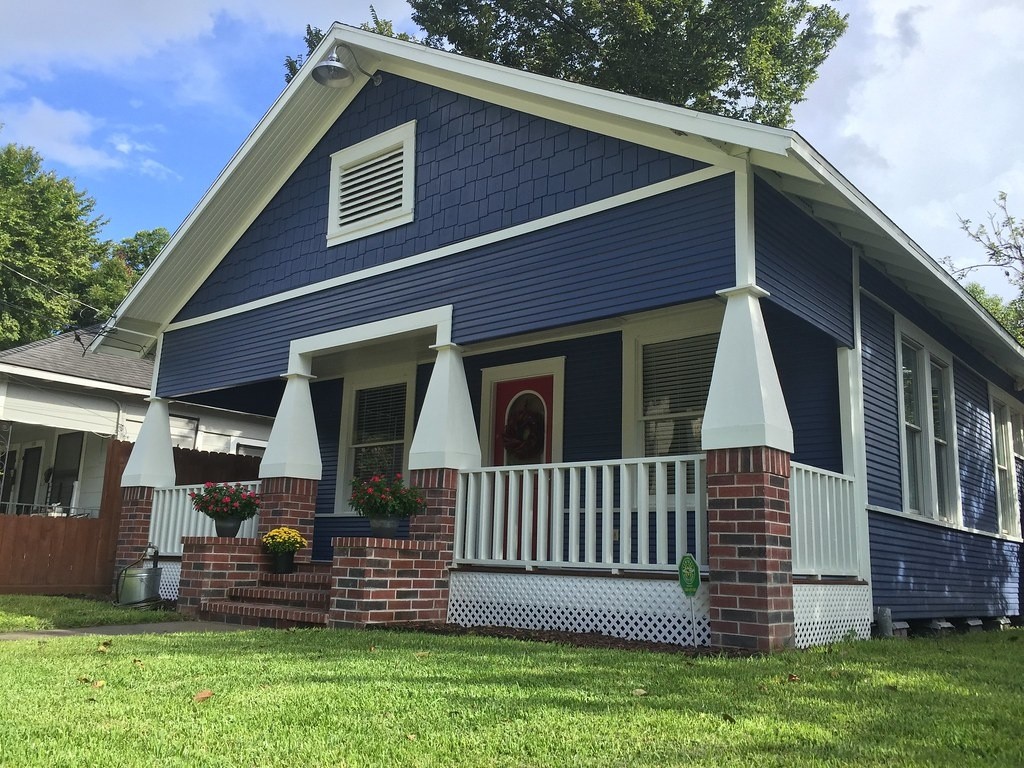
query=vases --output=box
[272,551,294,574]
[214,517,242,537]
[370,515,400,538]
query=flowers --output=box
[260,526,308,555]
[349,473,429,520]
[188,481,261,522]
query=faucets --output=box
[144,542,158,552]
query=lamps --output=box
[312,43,383,88]
